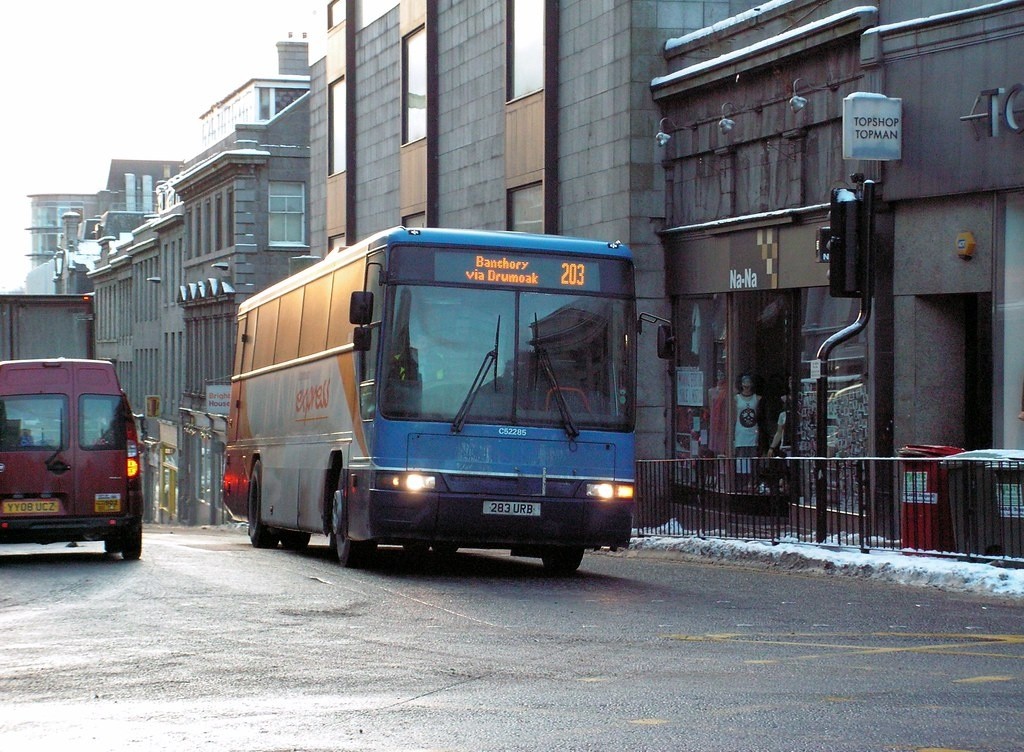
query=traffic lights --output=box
[825,186,860,298]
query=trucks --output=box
[0,356,143,561]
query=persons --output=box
[780,373,796,412]
[766,401,793,495]
[708,368,739,489]
[733,372,765,487]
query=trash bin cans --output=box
[948,447,1024,560]
[897,443,966,557]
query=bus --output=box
[221,224,677,581]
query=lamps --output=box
[719,102,740,135]
[789,77,839,114]
[655,118,681,147]
